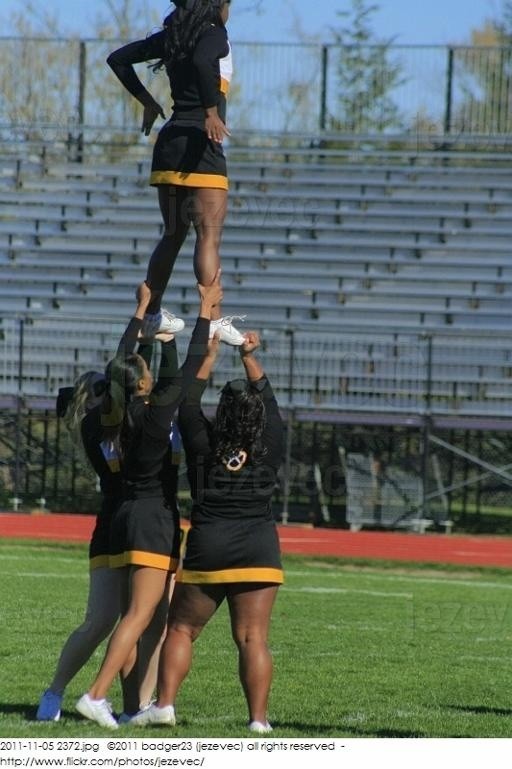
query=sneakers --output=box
[36,689,175,731]
[141,309,185,339]
[208,314,248,346]
[249,719,274,733]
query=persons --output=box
[104,2,258,350]
[123,324,287,735]
[36,278,179,724]
[72,258,224,729]
